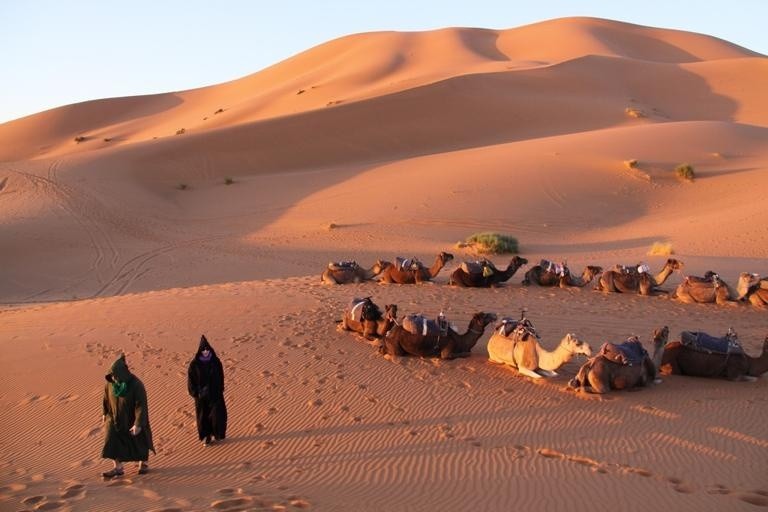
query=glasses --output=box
[201,346,210,351]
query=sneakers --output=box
[201,436,212,446]
[101,466,125,477]
[138,460,149,473]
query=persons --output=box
[187,333,228,447]
[100,352,157,478]
